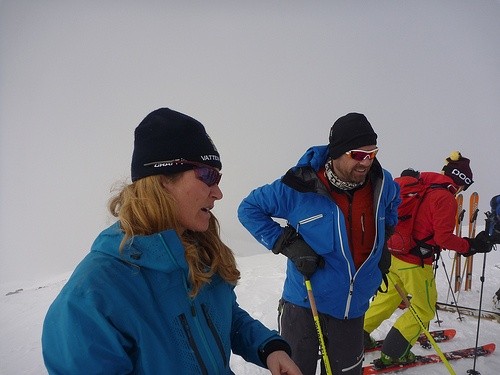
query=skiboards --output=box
[455,192,479,292]
[401,296,500,323]
[362,328,496,375]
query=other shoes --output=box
[379,347,417,365]
[364,336,377,350]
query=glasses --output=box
[194,168,222,186]
[346,147,379,161]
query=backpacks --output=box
[385,174,456,258]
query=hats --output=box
[130,107,222,181]
[328,112,378,160]
[442,151,475,190]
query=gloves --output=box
[457,231,493,258]
[271,223,326,276]
[379,224,395,274]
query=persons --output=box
[237,112,402,375]
[41,108,303,375]
[364,151,493,366]
[488,195,500,311]
